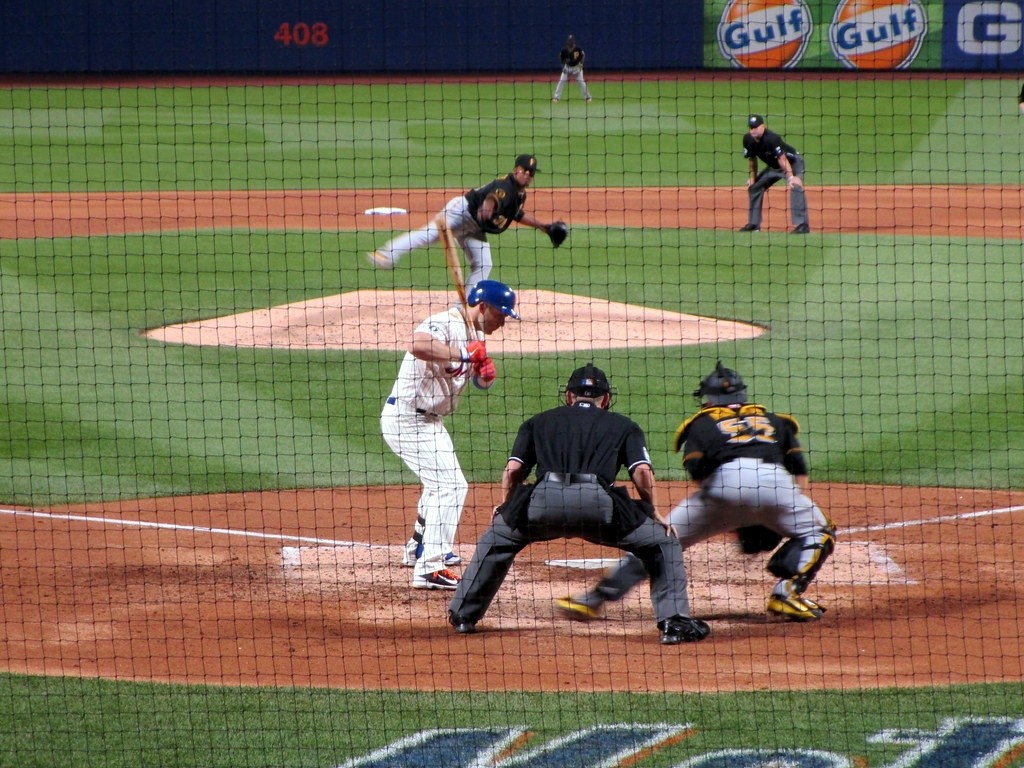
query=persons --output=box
[1018,83,1024,111]
[552,34,592,102]
[738,115,810,234]
[380,280,522,589]
[364,154,568,308]
[554,366,835,622]
[448,361,711,643]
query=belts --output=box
[541,471,600,484]
[387,397,439,418]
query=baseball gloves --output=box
[736,524,781,555]
[546,222,567,249]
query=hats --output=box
[566,362,610,398]
[697,360,748,405]
[515,154,537,172]
[747,114,764,129]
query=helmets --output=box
[567,35,575,41]
[467,279,522,321]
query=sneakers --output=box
[738,224,761,232]
[413,569,460,591]
[402,551,463,567]
[555,596,601,622]
[766,593,829,620]
[790,223,810,234]
[660,616,710,646]
[447,610,479,634]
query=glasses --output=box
[518,166,536,177]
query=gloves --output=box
[472,357,497,382]
[460,341,487,363]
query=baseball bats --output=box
[435,216,478,342]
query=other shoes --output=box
[366,251,392,271]
[585,96,592,101]
[552,97,560,102]
